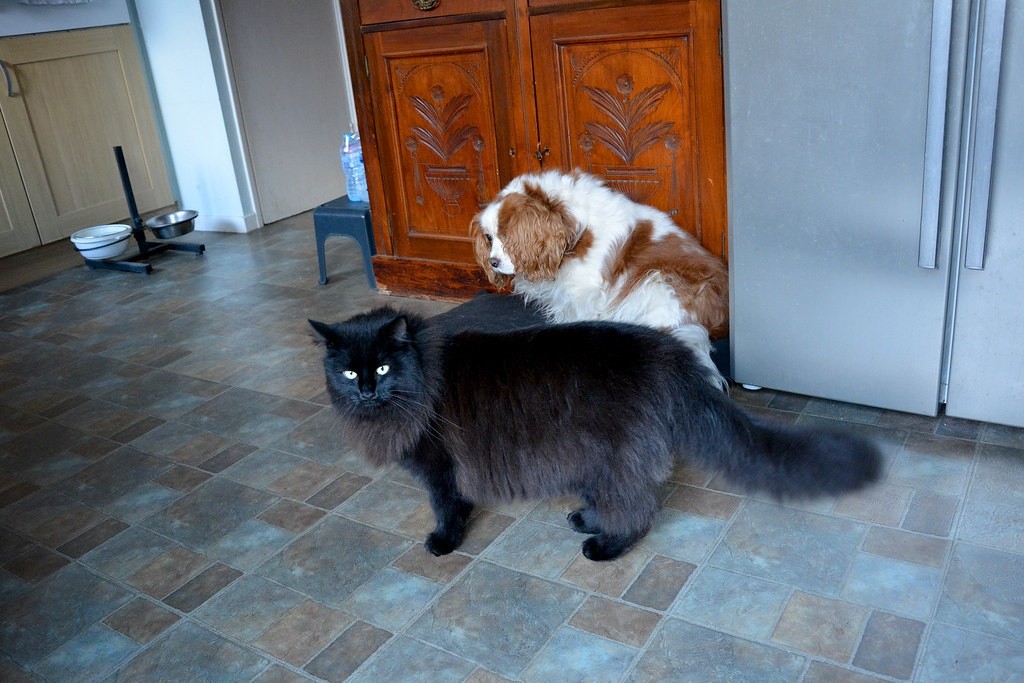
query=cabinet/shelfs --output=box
[338,0,728,302]
[0,24,178,291]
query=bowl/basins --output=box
[145,210,198,239]
[70,224,133,260]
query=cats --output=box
[307,302,883,562]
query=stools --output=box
[312,194,377,291]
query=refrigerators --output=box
[721,0,1024,427]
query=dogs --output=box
[468,166,734,398]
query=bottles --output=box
[340,133,367,201]
